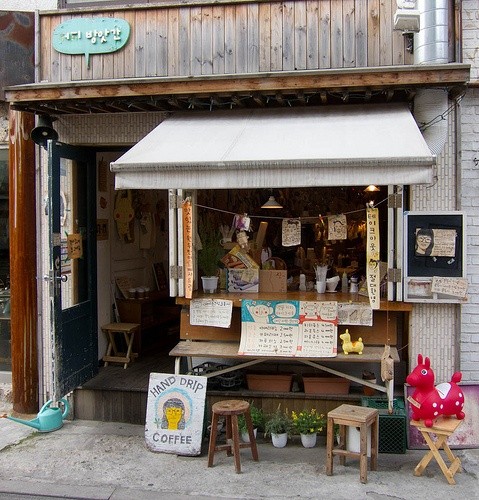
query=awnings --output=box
[109,103,437,188]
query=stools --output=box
[102,323,141,369]
[410,415,463,484]
[208,400,258,474]
[326,404,380,483]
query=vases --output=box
[300,433,316,447]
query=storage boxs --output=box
[302,373,350,395]
[362,398,407,454]
[246,370,295,392]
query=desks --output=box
[168,341,400,413]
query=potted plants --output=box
[335,424,340,444]
[238,400,294,448]
[200,246,219,294]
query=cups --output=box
[316,281,326,293]
[129,286,149,300]
[325,281,338,292]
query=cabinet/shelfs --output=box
[116,290,178,356]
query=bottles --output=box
[307,248,315,261]
[349,278,358,293]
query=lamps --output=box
[393,9,420,34]
[261,188,283,208]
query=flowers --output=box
[292,409,325,435]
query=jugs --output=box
[4,399,70,433]
